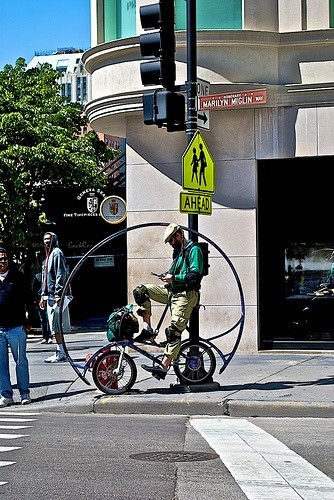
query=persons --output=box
[0,247,35,408]
[133,222,204,380]
[38,232,74,363]
[19,263,53,344]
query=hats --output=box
[163,223,181,244]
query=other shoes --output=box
[43,351,67,364]
[20,393,32,405]
[0,395,14,408]
[133,329,156,343]
[140,364,168,380]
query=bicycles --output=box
[91,284,217,396]
